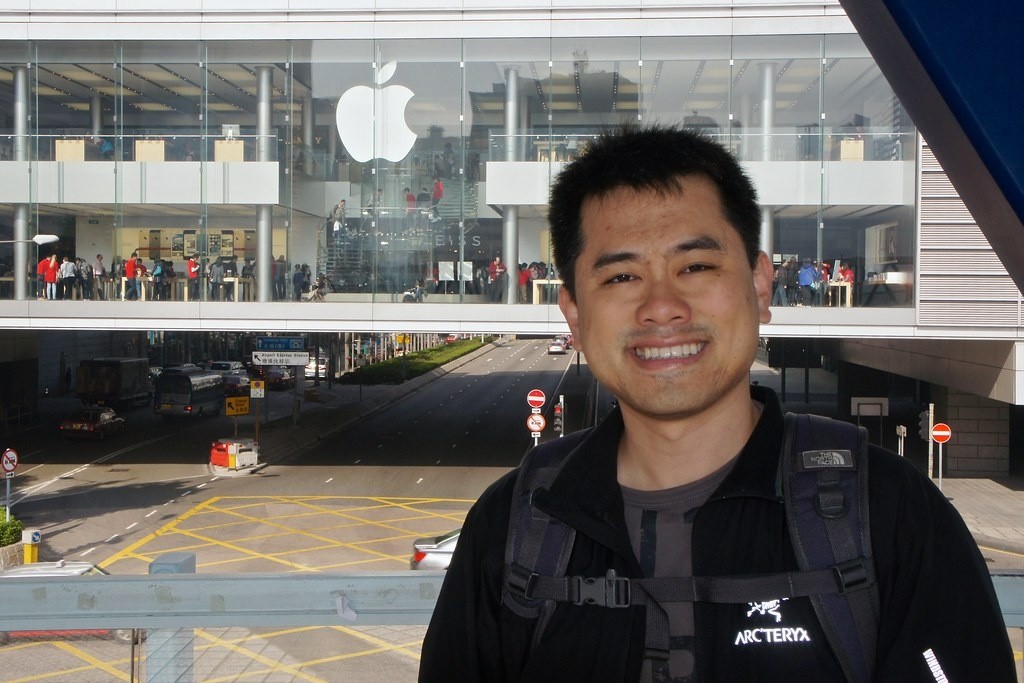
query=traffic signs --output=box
[225,396,250,416]
[256,336,304,351]
[252,352,310,366]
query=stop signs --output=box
[527,389,546,408]
[931,423,951,443]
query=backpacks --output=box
[81,268,93,280]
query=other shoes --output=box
[41,295,257,301]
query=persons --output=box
[435,143,455,179]
[413,280,423,303]
[773,256,855,307]
[272,255,331,303]
[564,130,578,162]
[418,130,1019,683]
[95,130,113,161]
[330,199,347,248]
[489,257,507,304]
[401,187,432,238]
[37,253,106,301]
[430,176,444,223]
[110,253,176,302]
[519,261,554,304]
[367,189,383,221]
[188,254,252,302]
[65,368,72,392]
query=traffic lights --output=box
[553,405,563,432]
[257,369,262,379]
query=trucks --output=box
[80,358,153,409]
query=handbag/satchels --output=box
[57,263,70,278]
[153,263,162,277]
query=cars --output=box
[447,333,463,343]
[223,377,251,396]
[410,528,461,570]
[148,362,203,376]
[0,560,146,645]
[59,405,128,441]
[265,370,294,390]
[547,335,573,354]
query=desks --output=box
[834,139,864,161]
[533,140,588,161]
[533,279,563,303]
[55,140,252,162]
[72,276,255,302]
[829,282,851,308]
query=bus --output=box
[154,371,225,420]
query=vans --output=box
[210,360,248,376]
[305,357,328,378]
